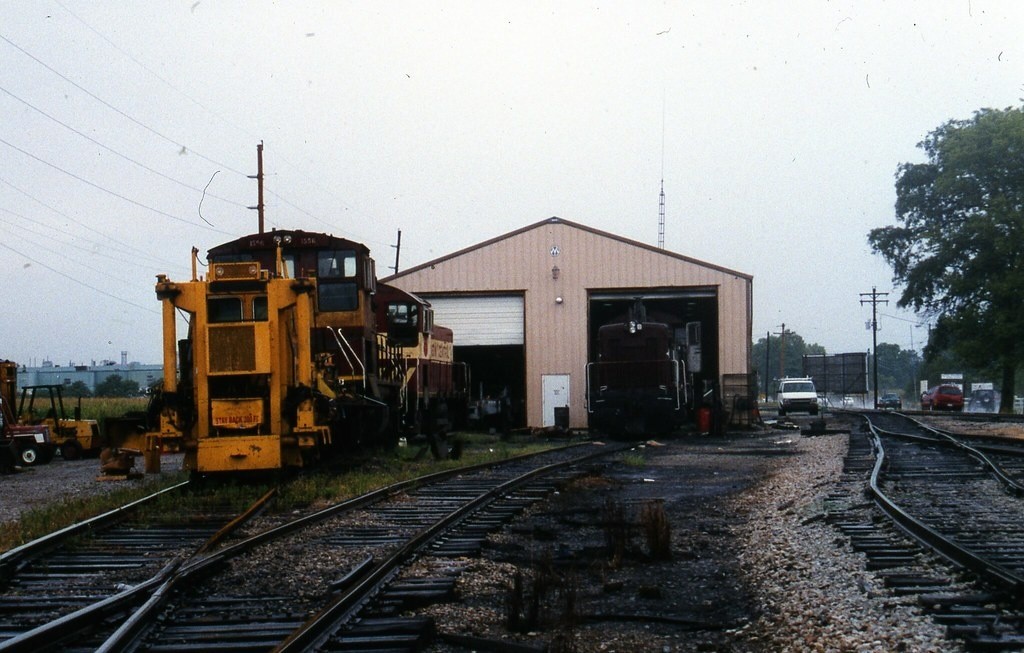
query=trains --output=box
[586,298,689,439]
[155,228,455,490]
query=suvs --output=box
[920,386,964,412]
[778,379,818,416]
[969,389,995,412]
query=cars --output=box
[880,392,902,410]
[816,396,828,406]
[840,397,853,407]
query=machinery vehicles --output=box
[0,395,52,467]
[0,358,102,461]
